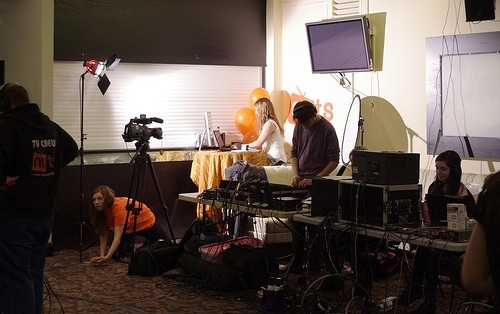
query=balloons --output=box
[243,131,262,150]
[249,87,269,109]
[234,106,256,136]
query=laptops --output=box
[425,194,462,227]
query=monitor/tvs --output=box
[305,17,372,74]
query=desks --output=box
[292,212,470,310]
[180,192,312,273]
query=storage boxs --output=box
[338,180,422,230]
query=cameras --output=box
[122,114,163,142]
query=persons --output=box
[284,101,341,275]
[458,170,500,314]
[392,150,474,314]
[318,145,383,296]
[90,185,171,266]
[230,98,288,166]
[0,82,78,314]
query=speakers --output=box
[352,150,420,185]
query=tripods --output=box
[115,143,178,263]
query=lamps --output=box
[78,53,121,263]
